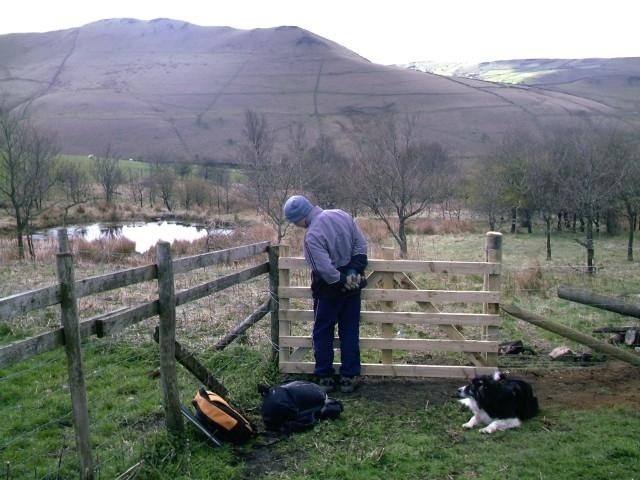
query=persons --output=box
[284,195,367,393]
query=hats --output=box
[284,196,313,221]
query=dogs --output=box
[455,371,539,435]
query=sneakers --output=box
[307,373,334,390]
[341,376,362,392]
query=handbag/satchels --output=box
[192,388,255,445]
[260,380,344,435]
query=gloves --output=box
[344,270,361,290]
